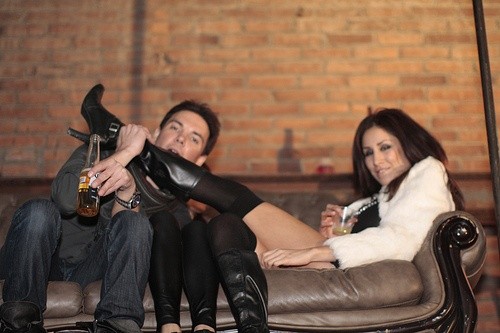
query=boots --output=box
[217,249,270,332]
[67,84,210,202]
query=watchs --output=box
[113,187,142,209]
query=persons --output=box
[68,83,457,333]
[146,161,220,333]
[0,100,220,333]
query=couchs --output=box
[0,211,487,333]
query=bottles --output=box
[319,157,334,173]
[75,134,101,217]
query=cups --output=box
[330,206,358,235]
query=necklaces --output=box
[352,196,379,215]
